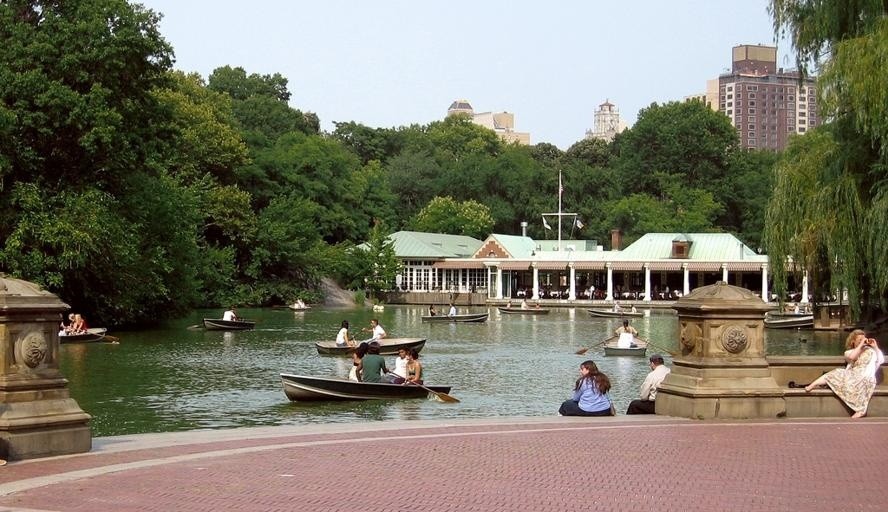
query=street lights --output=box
[520,222,527,236]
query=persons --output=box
[521,301,530,311]
[506,301,512,309]
[429,304,448,316]
[630,304,636,313]
[804,329,885,418]
[782,302,805,314]
[557,360,616,416]
[535,303,540,309]
[348,341,424,386]
[614,302,626,314]
[448,303,459,317]
[514,284,684,302]
[336,319,357,348]
[222,310,237,322]
[361,319,387,344]
[626,354,671,414]
[57,311,88,337]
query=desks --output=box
[771,291,813,301]
[514,286,682,300]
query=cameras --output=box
[865,338,872,344]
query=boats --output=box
[281,372,454,404]
[586,309,644,321]
[288,306,312,312]
[58,327,107,345]
[314,338,425,359]
[202,318,256,332]
[602,339,648,357]
[811,300,887,331]
[762,315,813,330]
[498,306,549,315]
[421,312,488,323]
